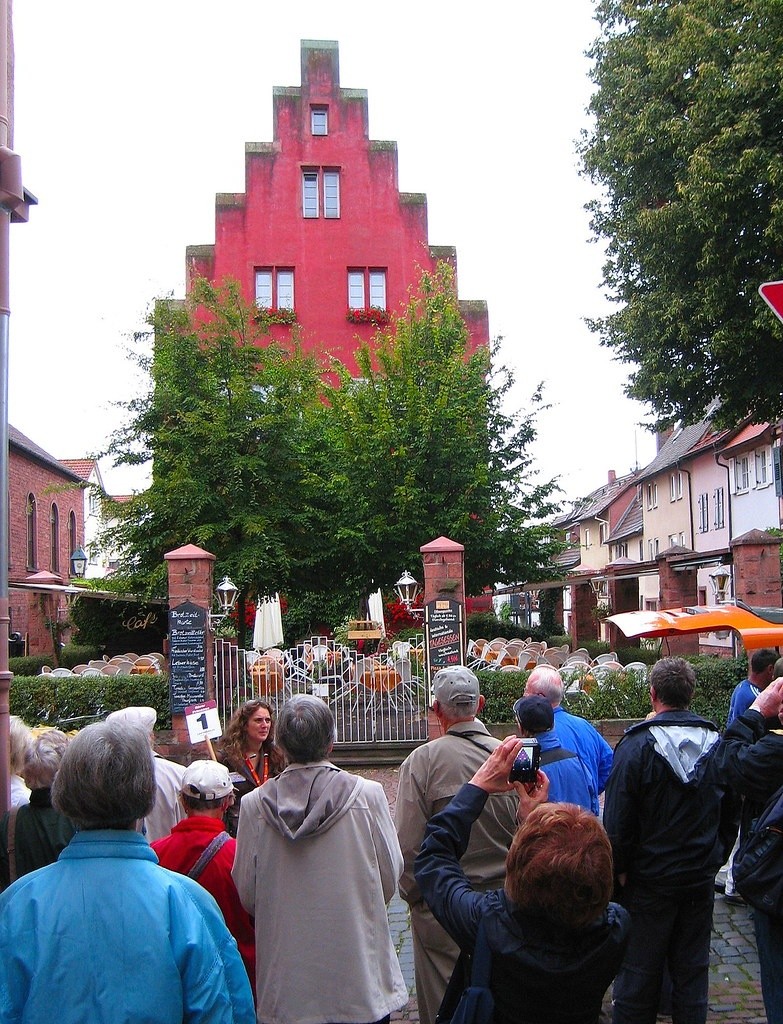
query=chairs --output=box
[35,653,165,677]
[245,640,427,715]
[467,637,648,718]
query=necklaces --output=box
[250,754,257,759]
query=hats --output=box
[106,707,157,733]
[181,760,234,801]
[431,665,480,707]
[513,696,554,732]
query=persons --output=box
[0,646,782,1024]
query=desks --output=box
[131,665,155,674]
[249,672,282,696]
[359,671,401,707]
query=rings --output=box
[537,784,542,788]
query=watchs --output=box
[749,706,766,720]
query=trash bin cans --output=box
[8,632,25,658]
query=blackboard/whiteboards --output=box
[426,596,463,711]
[169,599,209,714]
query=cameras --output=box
[509,739,541,782]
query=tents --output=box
[8,583,168,655]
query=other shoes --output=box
[725,895,748,906]
[715,885,726,894]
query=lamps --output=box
[590,577,608,600]
[707,561,736,607]
[208,575,238,633]
[70,541,87,579]
[393,570,424,627]
[57,584,80,612]
